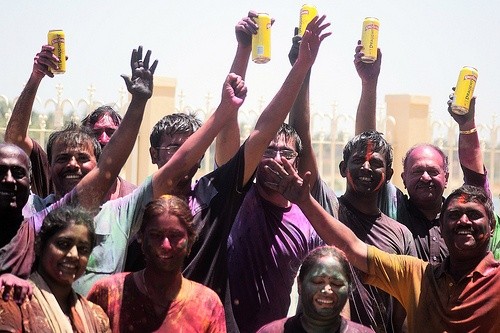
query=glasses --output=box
[262,145,298,160]
[154,144,179,156]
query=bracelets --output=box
[459,128,477,135]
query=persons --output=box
[0,45,248,300]
[86,195,227,333]
[124,14,332,332]
[446,86,495,203]
[0,206,112,333]
[212,10,324,333]
[288,26,419,333]
[262,156,500,333]
[253,245,375,333]
[354,38,452,268]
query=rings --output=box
[138,60,142,63]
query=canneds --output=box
[359,17,380,63]
[48,29,66,74]
[451,65,479,113]
[298,3,318,36]
[252,12,271,64]
